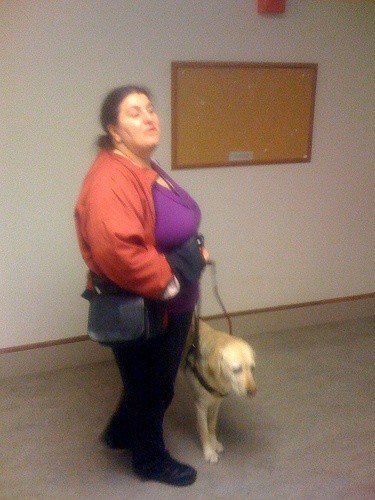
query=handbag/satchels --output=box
[81,274,150,346]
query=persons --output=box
[74,86,209,488]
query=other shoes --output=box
[132,451,197,487]
[104,432,129,450]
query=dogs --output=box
[180,316,257,464]
